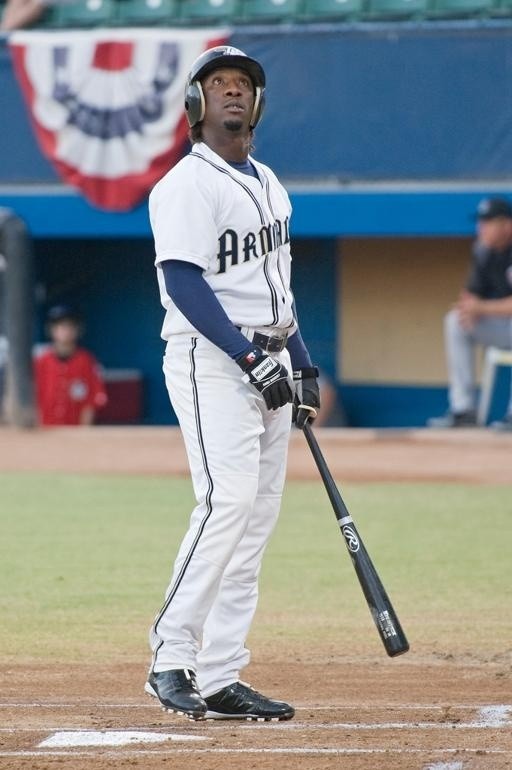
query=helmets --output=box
[183,46,267,130]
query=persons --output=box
[426,197,512,433]
[31,304,108,427]
[144,45,321,723]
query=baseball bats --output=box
[303,421,407,656]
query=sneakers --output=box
[196,682,294,723]
[144,668,207,717]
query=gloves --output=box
[237,346,295,412]
[291,365,320,430]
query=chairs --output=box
[471,345,512,428]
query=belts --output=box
[237,327,289,353]
